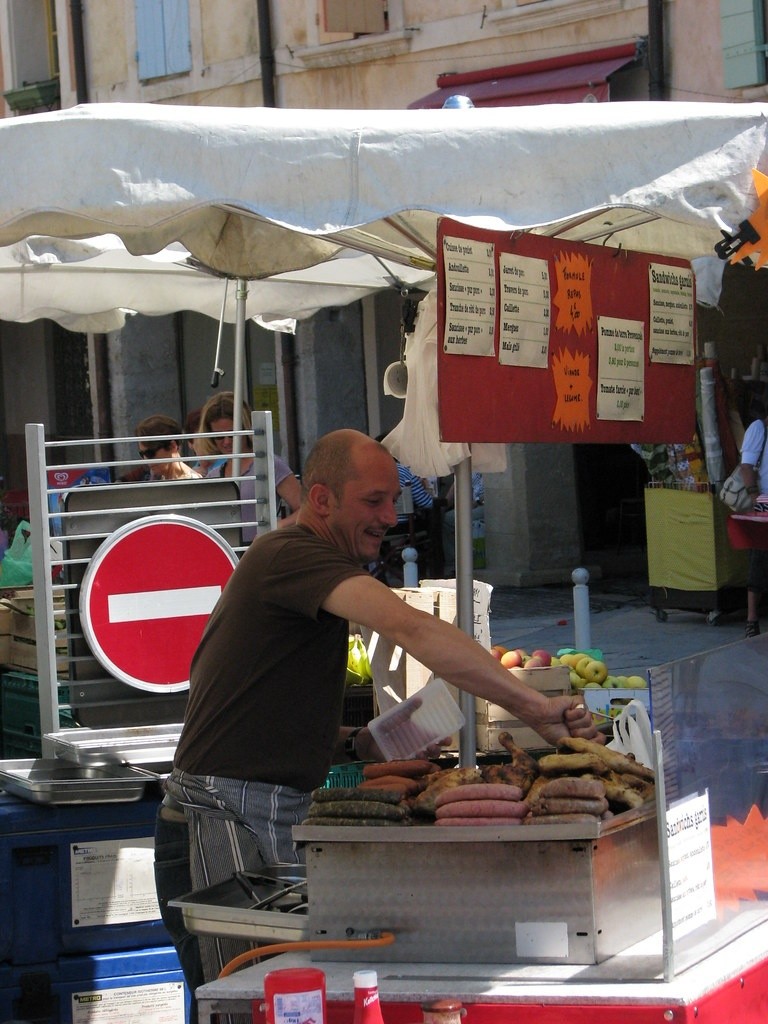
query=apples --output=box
[550,653,647,688]
[490,644,552,671]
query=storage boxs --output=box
[0,585,70,678]
[578,687,649,725]
[473,664,572,752]
[320,761,368,789]
[0,793,194,1024]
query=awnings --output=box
[407,47,645,104]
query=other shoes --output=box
[745,621,760,639]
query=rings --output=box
[575,704,586,709]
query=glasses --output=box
[139,446,166,459]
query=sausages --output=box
[301,759,614,826]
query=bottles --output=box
[263,968,328,1024]
[352,970,385,1024]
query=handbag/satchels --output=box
[719,419,768,513]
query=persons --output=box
[374,433,485,580]
[137,407,225,480]
[198,390,303,544]
[728,418,768,636]
[156,427,607,1024]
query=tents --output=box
[1,98,768,790]
